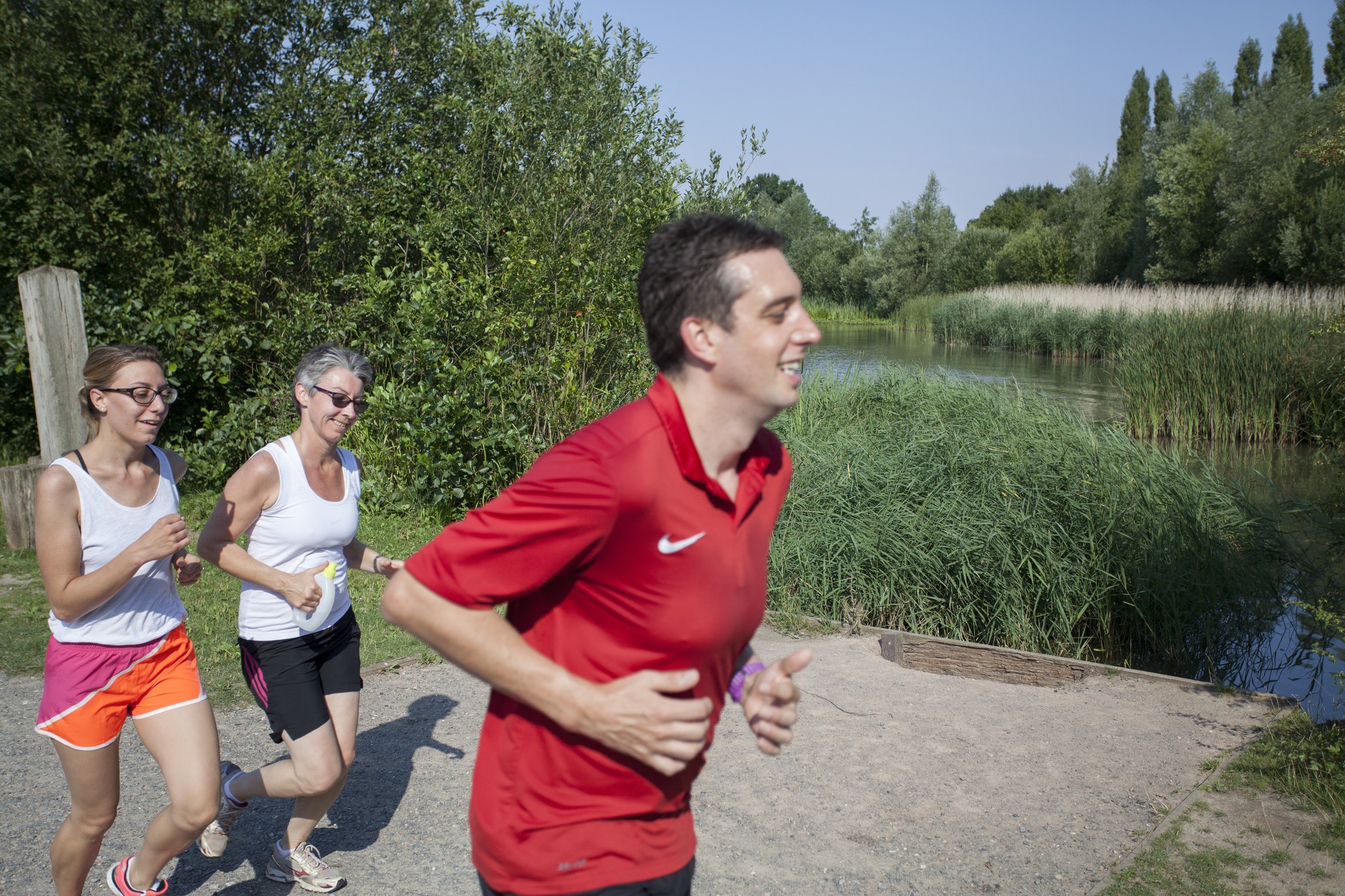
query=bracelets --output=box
[373,555,384,574]
[728,662,767,703]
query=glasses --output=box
[313,385,370,414]
[99,386,180,405]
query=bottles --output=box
[292,562,337,632]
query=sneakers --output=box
[265,836,347,893]
[106,855,167,896]
[195,760,248,858]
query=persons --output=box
[34,343,222,896]
[195,344,406,895]
[381,210,824,895]
